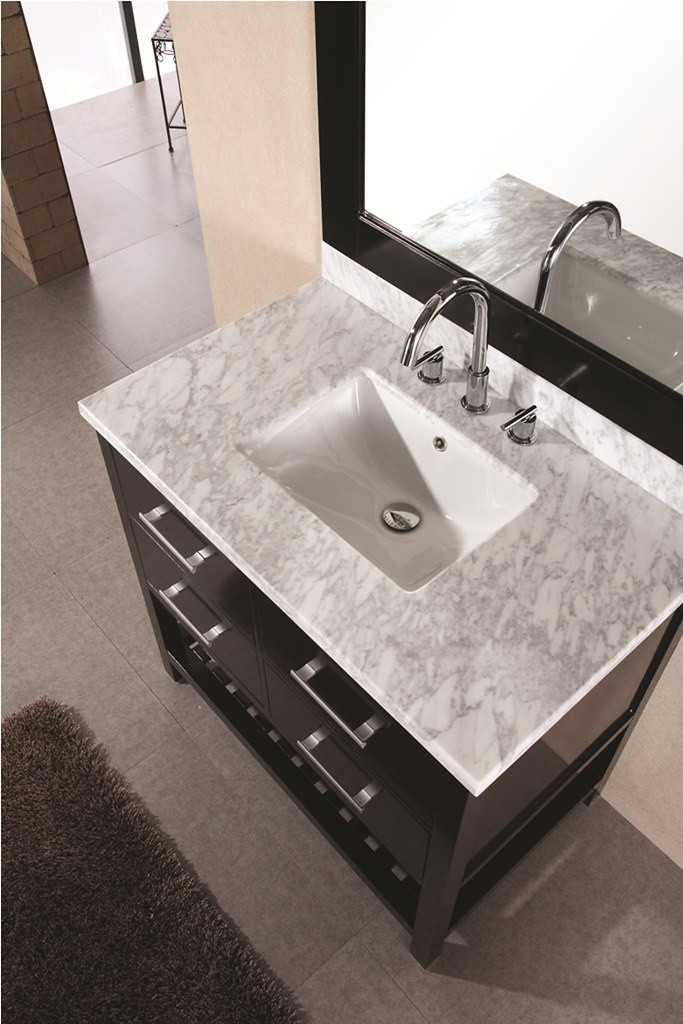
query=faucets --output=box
[399,276,493,415]
[532,199,622,316]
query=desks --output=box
[152,13,188,150]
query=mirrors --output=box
[313,0,683,464]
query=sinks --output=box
[234,363,541,594]
[488,239,683,392]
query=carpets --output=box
[1,698,305,1024]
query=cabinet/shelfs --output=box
[95,433,682,972]
